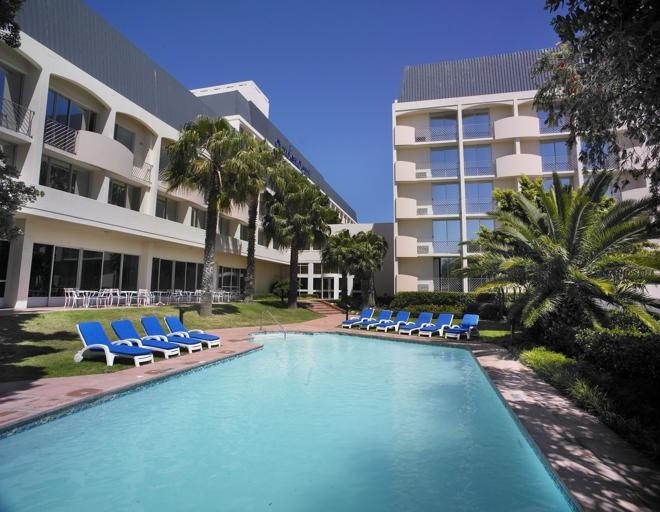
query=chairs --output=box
[340,307,481,343]
[72,314,221,368]
[62,285,242,309]
